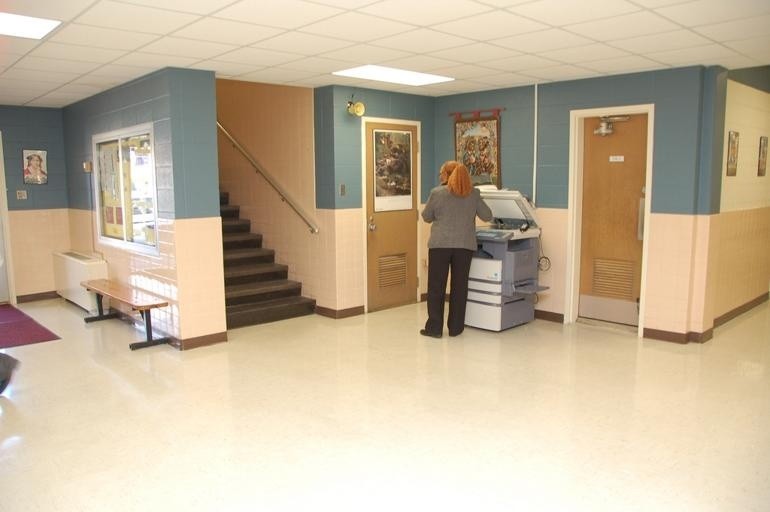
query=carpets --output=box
[0,303,62,348]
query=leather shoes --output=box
[421,330,442,338]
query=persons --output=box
[419,160,493,340]
[23,154,46,179]
[476,134,495,175]
[459,136,479,174]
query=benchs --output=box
[79,278,170,351]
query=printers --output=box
[465,189,552,333]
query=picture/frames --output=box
[454,116,501,190]
